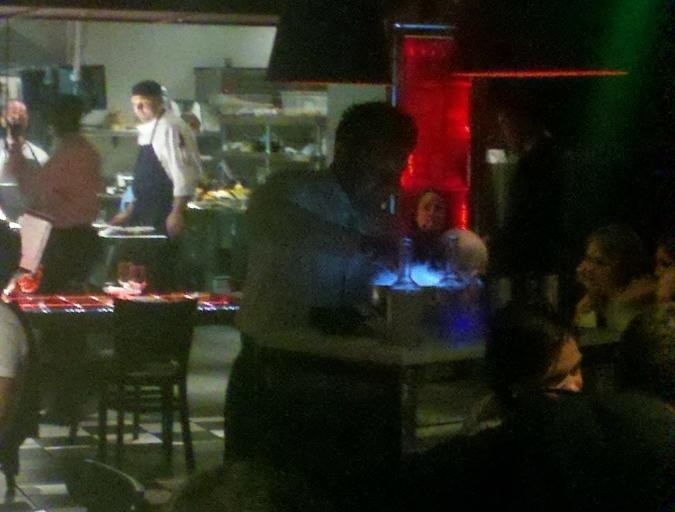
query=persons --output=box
[407,185,452,248]
[433,227,489,292]
[489,299,583,420]
[0,99,51,228]
[0,223,30,440]
[608,232,675,351]
[7,94,109,422]
[109,81,195,287]
[222,101,417,512]
[605,303,674,401]
[570,225,638,329]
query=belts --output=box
[116,262,134,291]
[128,265,147,302]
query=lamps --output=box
[82,459,145,512]
[98,298,201,462]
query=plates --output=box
[218,115,323,191]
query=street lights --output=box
[19,206,55,272]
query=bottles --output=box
[132,80,163,96]
